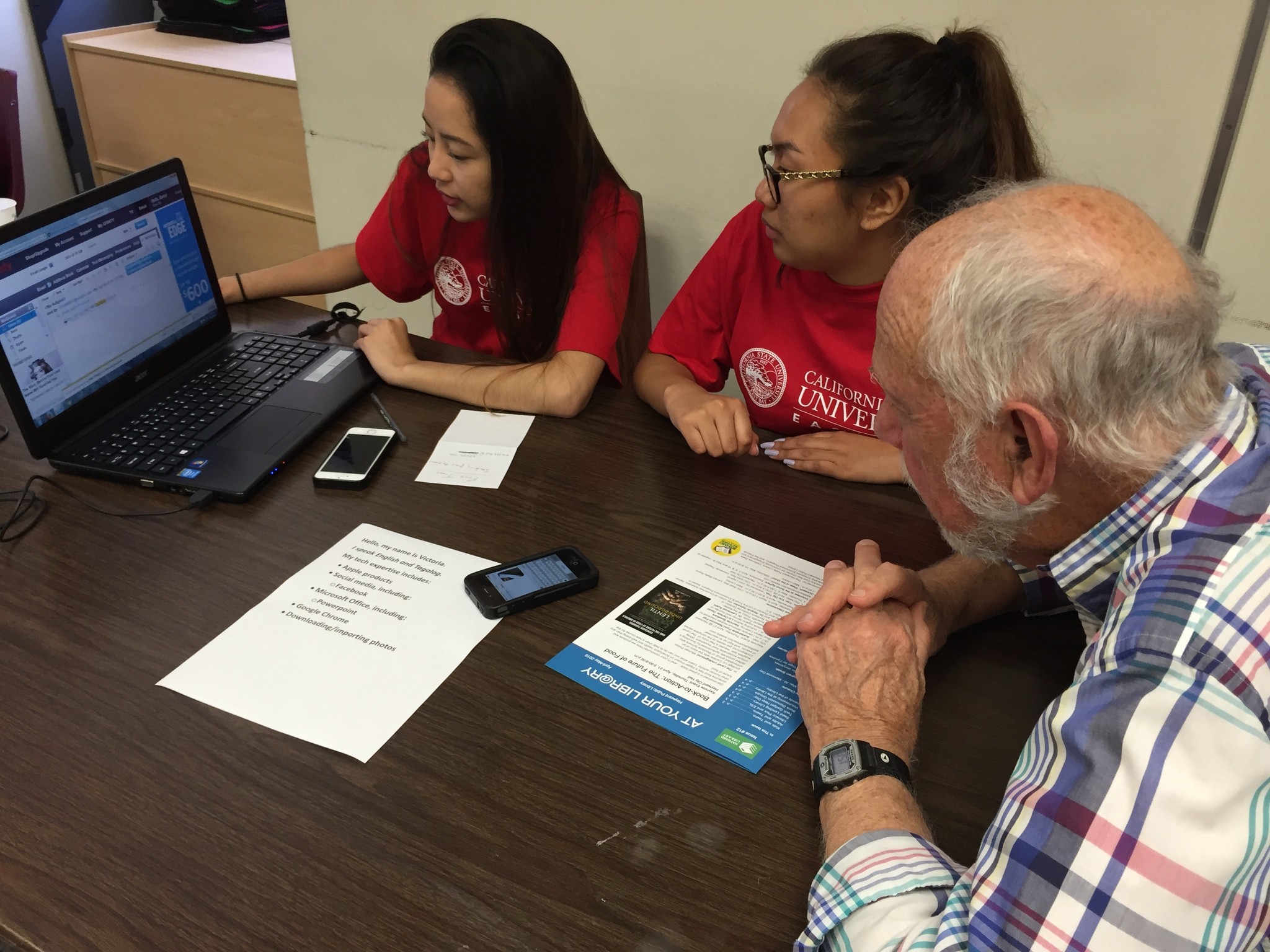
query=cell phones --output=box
[312,427,399,489]
[463,546,600,619]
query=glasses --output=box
[758,138,916,207]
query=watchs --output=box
[810,739,911,797]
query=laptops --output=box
[0,159,380,502]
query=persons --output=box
[632,29,1044,484]
[217,18,641,418]
[765,179,1270,952]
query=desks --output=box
[0,293,1085,952]
[64,19,327,311]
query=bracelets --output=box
[235,273,248,301]
